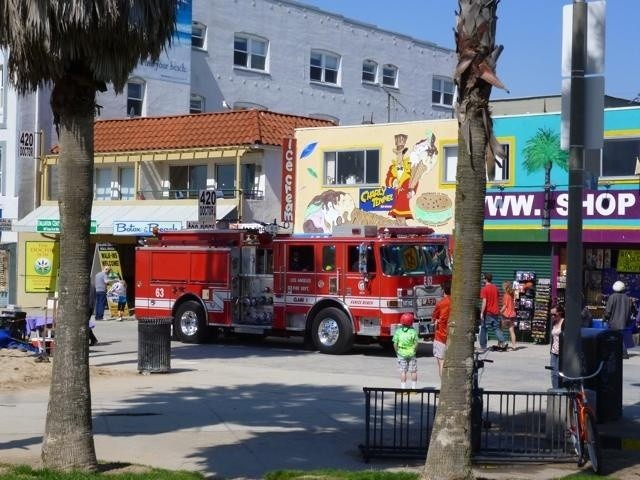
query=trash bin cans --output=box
[558,328,624,424]
[138,317,173,374]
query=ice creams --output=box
[303,189,407,233]
[407,129,439,189]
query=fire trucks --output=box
[132,221,453,359]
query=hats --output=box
[613,280,626,293]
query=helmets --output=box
[401,312,414,327]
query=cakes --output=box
[414,191,453,225]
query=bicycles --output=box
[539,358,617,477]
[472,343,510,456]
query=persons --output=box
[472,271,509,355]
[111,278,128,321]
[93,265,114,320]
[548,305,564,391]
[604,279,632,359]
[391,313,420,395]
[493,280,516,350]
[89,277,99,347]
[430,279,451,398]
